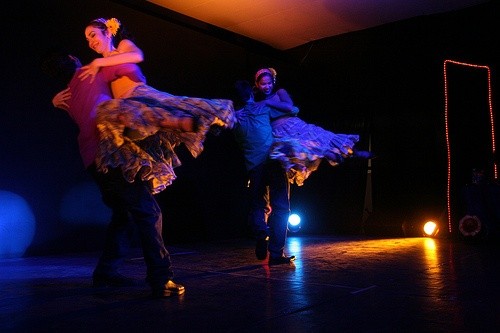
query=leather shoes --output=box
[152,280,186,297]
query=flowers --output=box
[105,17,121,37]
[268,68,277,78]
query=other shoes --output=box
[254,239,268,260]
[267,253,296,267]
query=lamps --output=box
[287,214,301,233]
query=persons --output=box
[50,17,236,292]
[229,66,374,264]
